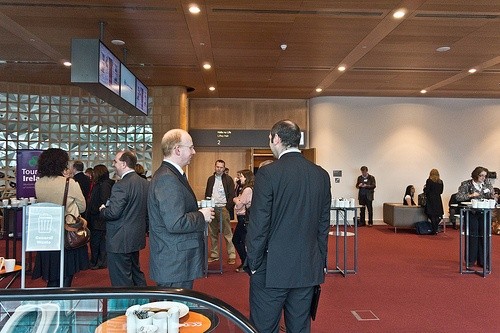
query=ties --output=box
[183,172,189,186]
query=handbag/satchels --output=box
[353,217,366,226]
[415,221,434,234]
[63,177,91,250]
[243,206,251,231]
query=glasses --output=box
[174,145,195,151]
[479,175,488,179]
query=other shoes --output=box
[227,259,236,265]
[207,257,219,262]
[358,224,366,227]
[478,264,489,269]
[235,266,246,273]
[88,264,107,270]
[453,226,457,230]
[465,261,474,268]
[369,224,372,227]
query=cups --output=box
[471,199,496,208]
[125,304,180,332]
[330,198,356,208]
[197,198,215,207]
[4,258,16,271]
[3,197,35,207]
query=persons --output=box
[403,184,416,206]
[232,169,255,273]
[87,164,113,270]
[245,120,332,333]
[32,148,89,287]
[99,149,148,287]
[70,160,89,198]
[84,167,94,200]
[147,128,216,289]
[492,188,500,206]
[456,166,492,269]
[223,167,230,176]
[356,165,376,227]
[133,163,148,180]
[259,159,274,169]
[449,193,467,230]
[425,169,445,234]
[235,170,242,197]
[204,160,237,265]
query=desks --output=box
[198,204,226,279]
[324,204,366,279]
[0,203,31,266]
[451,203,500,278]
[92,306,220,333]
[0,264,21,290]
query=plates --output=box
[142,300,190,318]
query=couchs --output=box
[382,202,427,234]
